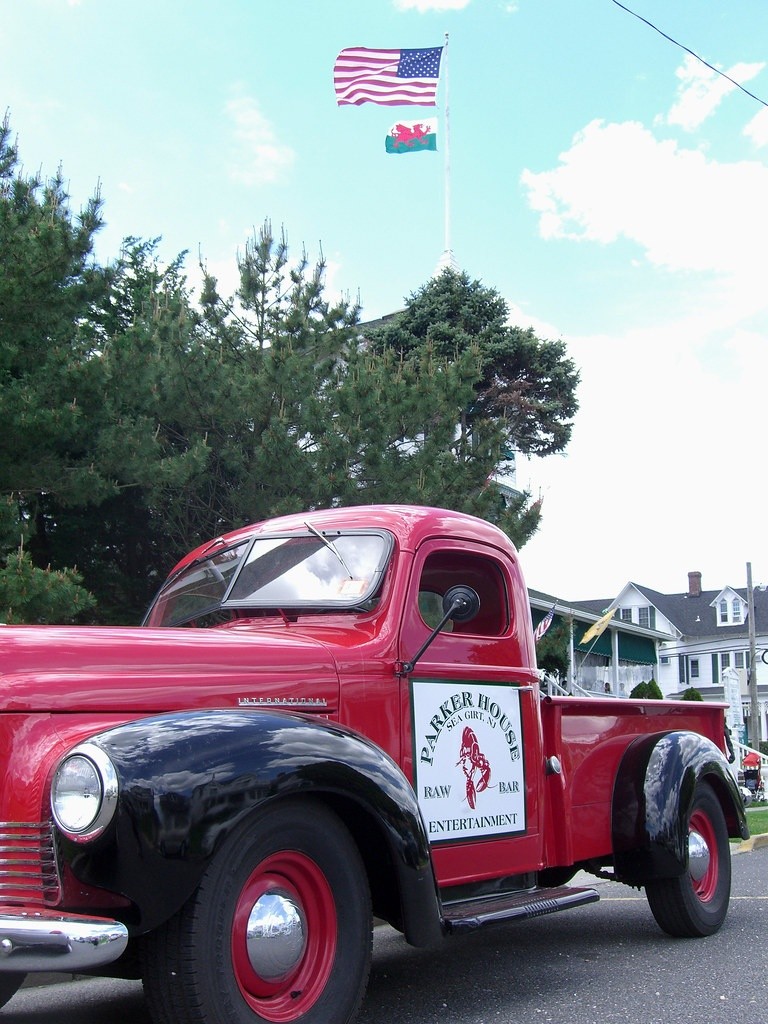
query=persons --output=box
[540,680,625,696]
[723,723,736,763]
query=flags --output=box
[534,603,555,643]
[385,117,438,154]
[580,609,617,644]
[333,46,444,107]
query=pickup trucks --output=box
[1,502,749,1023]
